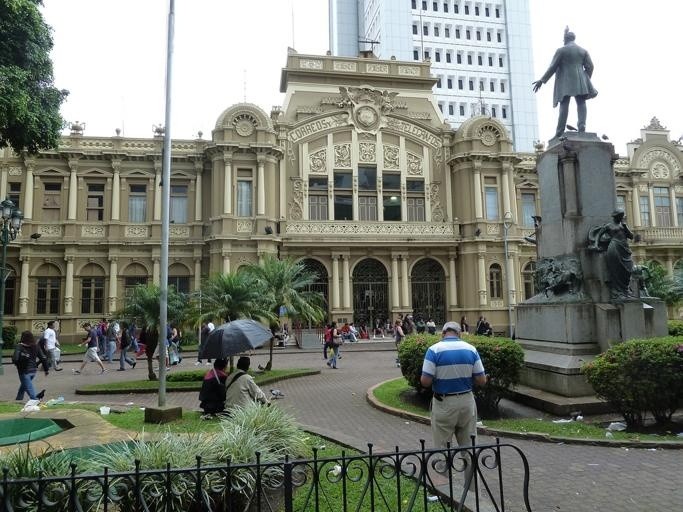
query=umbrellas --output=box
[197,315,273,373]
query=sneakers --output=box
[132,362,136,369]
[332,367,339,369]
[154,366,170,371]
[206,362,212,366]
[116,367,125,371]
[194,360,204,366]
[326,362,332,368]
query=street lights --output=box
[501,212,515,341]
[0,196,22,375]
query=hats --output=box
[442,321,462,334]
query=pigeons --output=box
[396,359,400,367]
[258,364,264,370]
[35,389,46,401]
[269,389,284,398]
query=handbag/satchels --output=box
[396,328,401,342]
[332,337,343,345]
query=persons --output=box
[198,357,230,412]
[72,314,182,374]
[533,31,597,138]
[322,313,494,369]
[13,330,48,399]
[36,320,61,376]
[199,321,211,362]
[45,321,63,372]
[224,356,272,413]
[279,323,290,344]
[595,207,633,299]
[420,321,487,492]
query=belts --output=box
[432,390,473,402]
